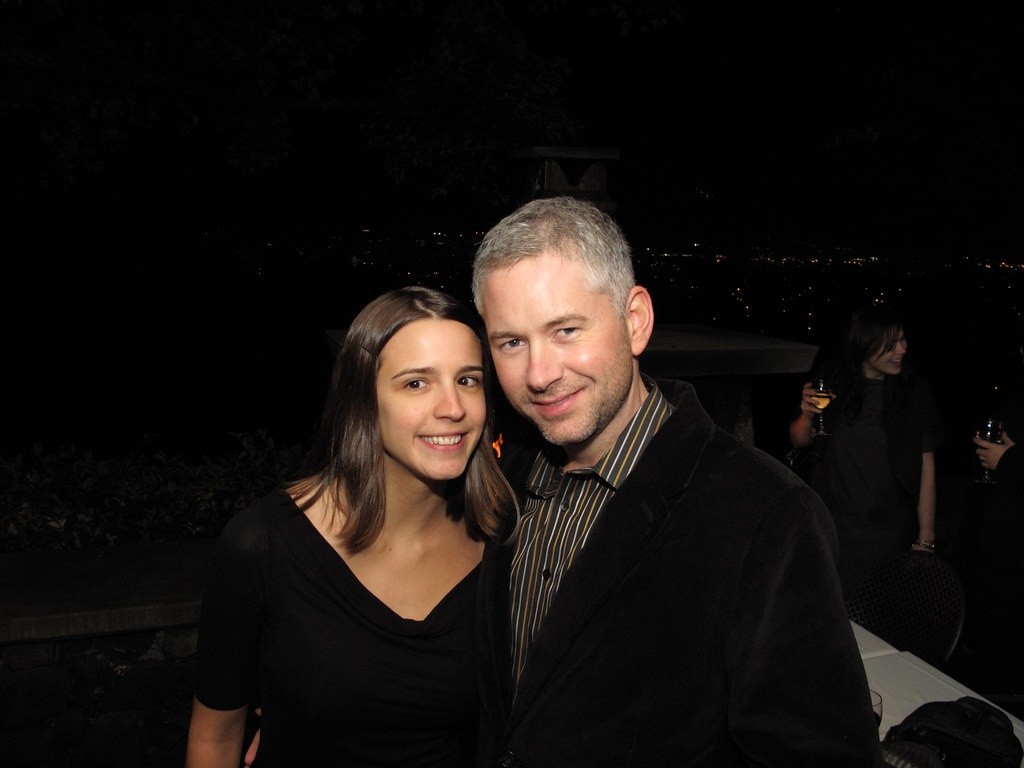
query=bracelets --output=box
[914,538,934,549]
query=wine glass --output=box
[808,378,833,436]
[976,419,1004,484]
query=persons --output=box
[187,285,521,768]
[959,397,1024,719]
[783,298,936,592]
[469,197,885,767]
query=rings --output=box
[978,454,984,461]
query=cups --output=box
[870,689,882,728]
